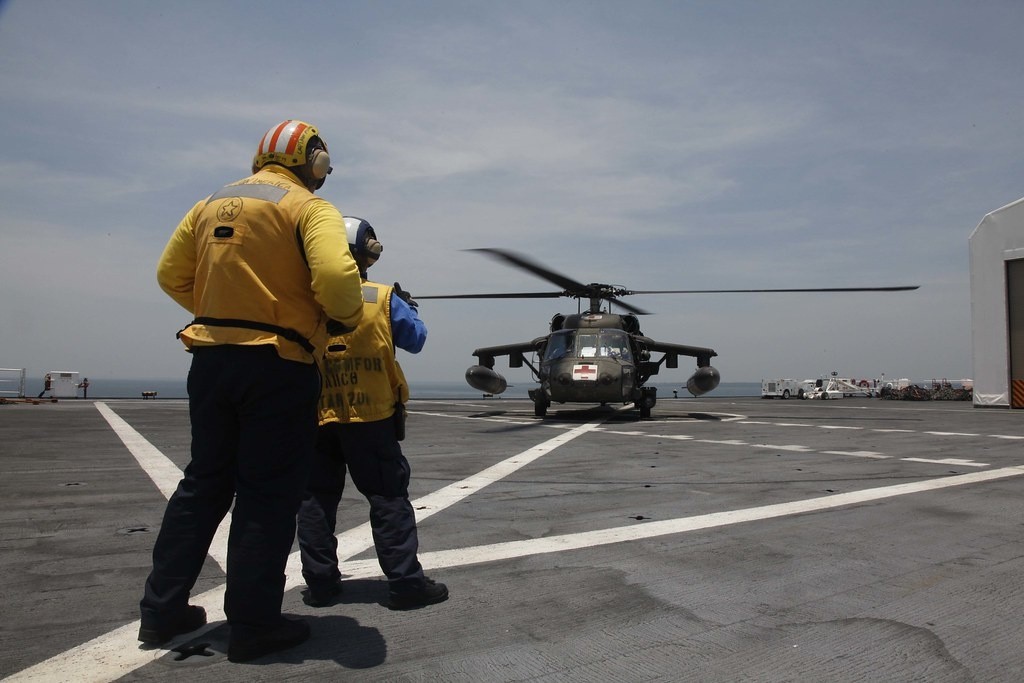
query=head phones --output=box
[362,237,382,268]
[251,139,331,181]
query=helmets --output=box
[254,119,330,191]
[342,215,377,266]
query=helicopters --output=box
[410,247,922,422]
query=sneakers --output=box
[307,579,341,607]
[387,576,448,610]
[228,616,311,662]
[138,605,206,643]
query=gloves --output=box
[394,282,418,307]
[326,319,358,337]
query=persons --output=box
[296,214,449,610]
[136,117,368,662]
